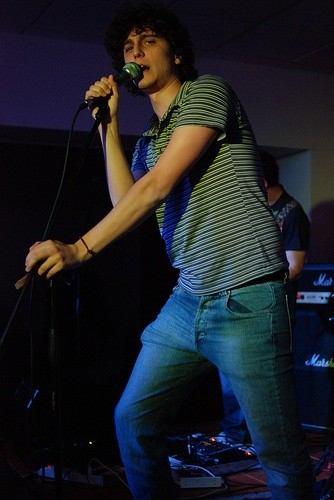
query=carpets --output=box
[0,415,334,500]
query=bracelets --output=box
[79,236,97,256]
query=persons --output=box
[203,151,310,463]
[26,2,314,500]
